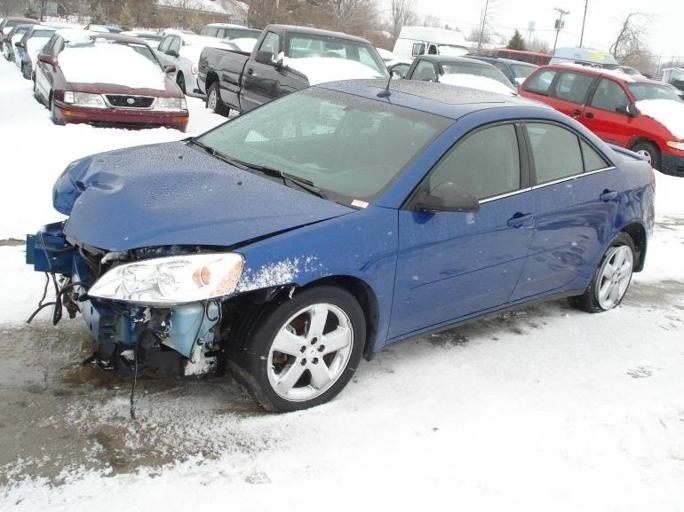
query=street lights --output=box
[552,7,571,55]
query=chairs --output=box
[356,116,571,197]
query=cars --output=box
[24,79,655,414]
